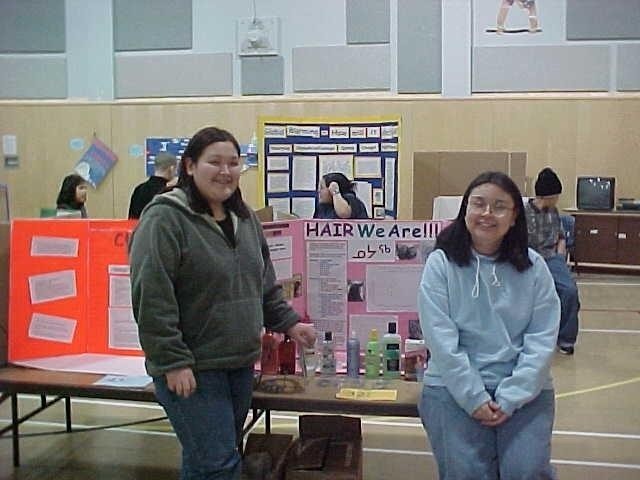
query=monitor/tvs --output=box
[576,176,615,210]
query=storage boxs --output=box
[290,411,366,480]
[241,431,293,479]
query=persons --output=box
[55,173,89,219]
[311,172,370,220]
[415,172,560,480]
[128,151,178,219]
[524,168,583,356]
[127,126,320,480]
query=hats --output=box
[535,168,562,196]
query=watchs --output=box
[331,188,339,195]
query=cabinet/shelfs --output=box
[562,204,640,273]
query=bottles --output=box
[365,329,381,379]
[381,321,402,381]
[278,334,296,375]
[346,329,361,382]
[261,327,279,375]
[321,330,337,375]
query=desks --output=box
[0,366,426,468]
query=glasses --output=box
[466,194,515,217]
